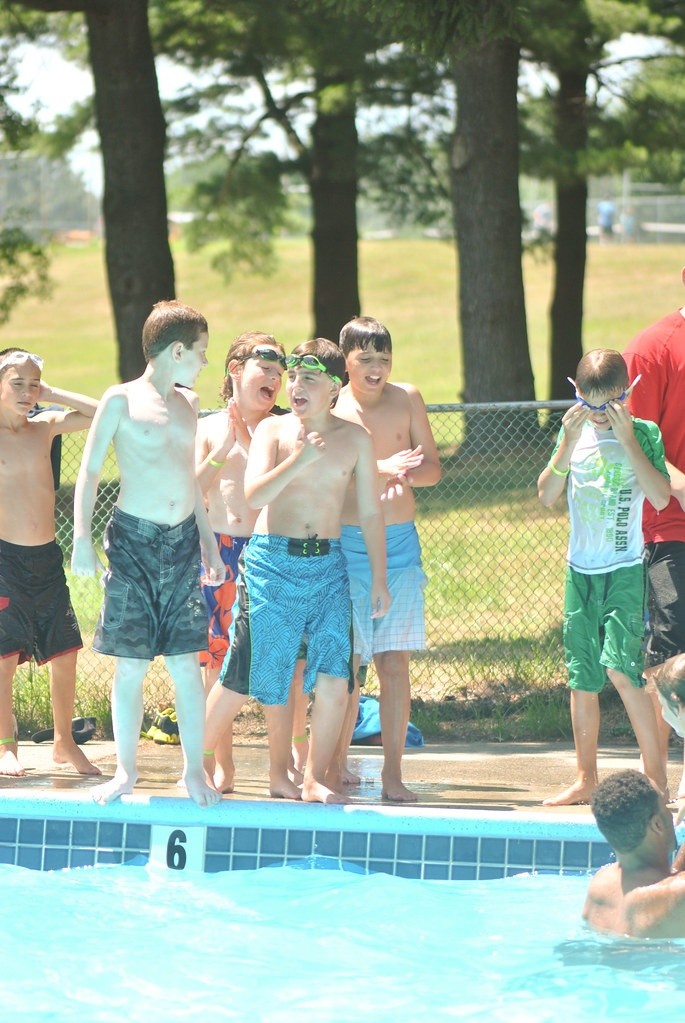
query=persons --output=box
[195,330,304,801]
[0,348,103,778]
[330,316,443,802]
[642,653,684,830]
[202,337,393,806]
[595,192,645,246]
[619,267,684,788]
[69,296,227,811]
[581,768,684,939]
[536,349,672,808]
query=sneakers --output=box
[153,711,180,745]
[146,707,175,739]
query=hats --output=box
[31,717,96,744]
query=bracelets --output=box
[549,457,571,476]
[207,451,227,467]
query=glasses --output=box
[1,351,44,373]
[567,375,640,414]
[285,354,341,384]
[238,348,287,371]
